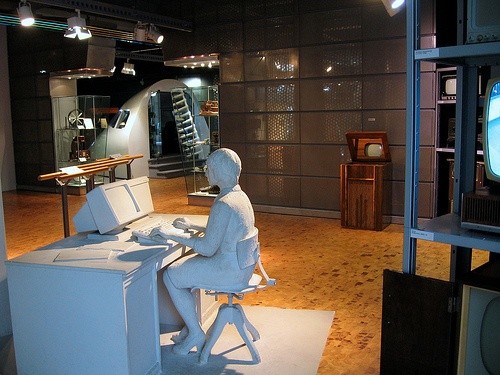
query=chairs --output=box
[200,227,276,366]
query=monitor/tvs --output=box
[72,181,143,234]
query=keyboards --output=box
[132,219,188,241]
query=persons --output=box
[150,148,256,354]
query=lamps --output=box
[131,20,164,44]
[15,0,35,27]
[120,58,136,76]
[381,0,408,16]
[63,8,93,41]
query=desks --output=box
[4,213,209,375]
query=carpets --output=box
[159,305,336,375]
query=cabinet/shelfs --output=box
[170,84,220,197]
[402,0,500,288]
[339,130,392,232]
[52,94,110,186]
